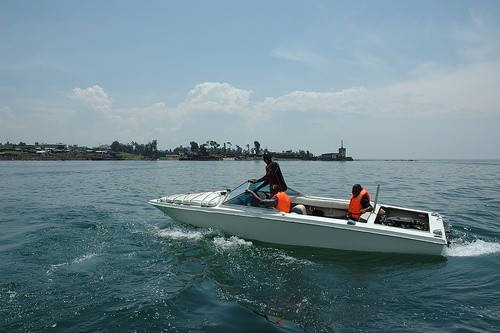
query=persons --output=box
[248,153,287,198]
[247,184,291,213]
[328,184,372,222]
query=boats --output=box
[147,177,450,256]
